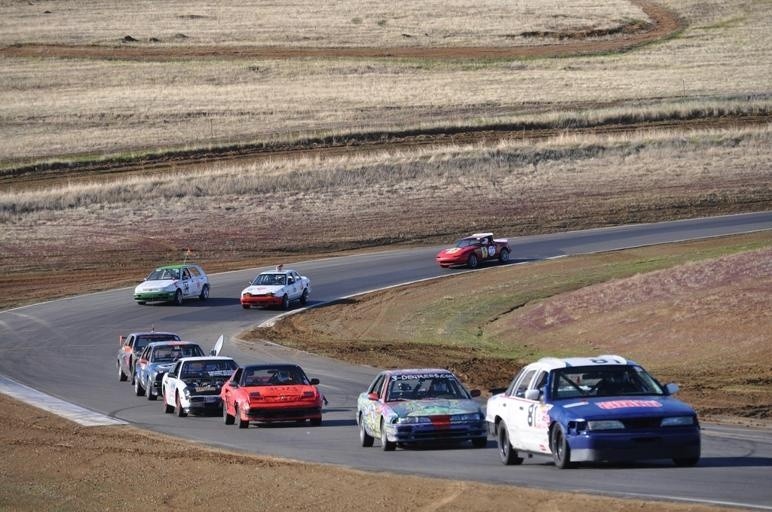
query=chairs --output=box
[271,371,291,383]
[430,381,450,398]
[598,373,642,395]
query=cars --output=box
[220,364,324,429]
[117,332,181,385]
[355,368,490,451]
[161,356,241,417]
[133,264,209,306]
[435,232,512,269]
[240,270,312,310]
[133,340,206,401]
[484,353,701,470]
